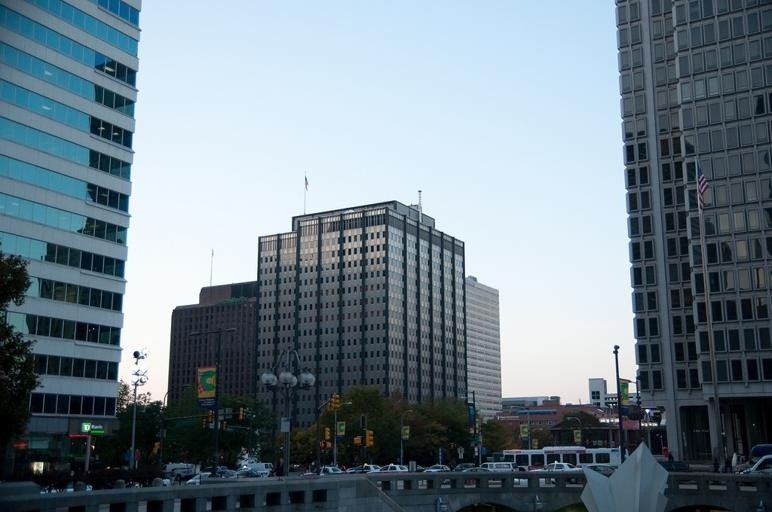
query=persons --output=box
[163,476,168,487]
[125,480,137,488]
[165,475,172,487]
[662,443,669,458]
[170,468,176,484]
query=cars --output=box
[40,480,93,494]
[185,462,276,485]
[319,460,617,485]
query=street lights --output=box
[261,346,316,476]
[159,383,194,462]
[333,401,353,467]
[400,409,414,464]
[507,405,531,449]
[612,345,625,463]
[207,326,238,432]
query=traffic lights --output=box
[328,392,340,411]
[324,427,330,440]
[365,430,374,448]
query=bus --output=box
[502,446,629,471]
